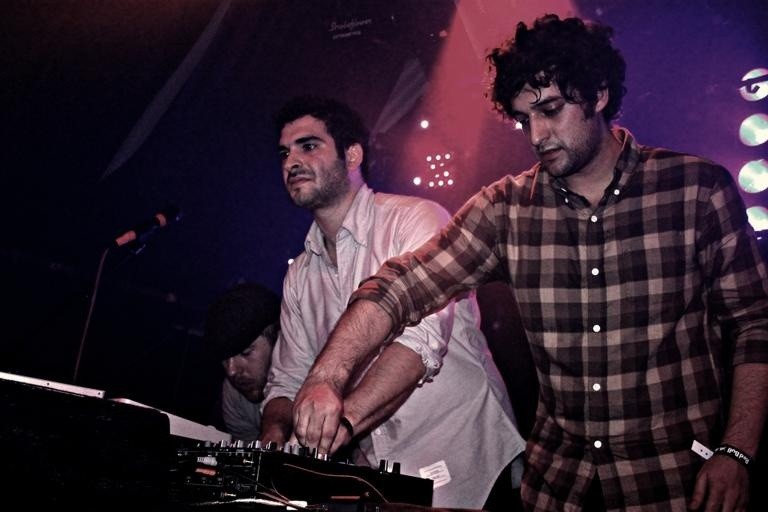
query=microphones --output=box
[115,213,167,246]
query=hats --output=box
[202,280,275,364]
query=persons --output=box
[201,282,365,464]
[258,95,527,512]
[292,14,767,512]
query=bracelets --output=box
[711,445,751,469]
[340,417,356,440]
